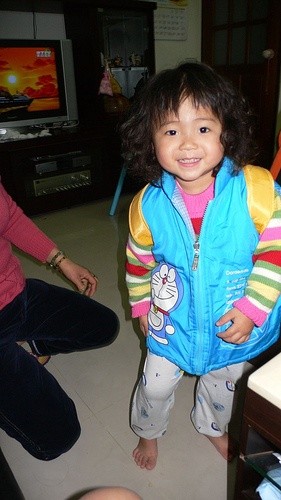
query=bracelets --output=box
[49,250,63,268]
[54,255,68,271]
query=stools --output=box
[109,150,137,216]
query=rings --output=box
[93,275,95,278]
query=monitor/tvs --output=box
[0,39,79,134]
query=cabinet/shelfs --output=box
[0,130,113,217]
[65,7,155,191]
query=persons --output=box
[78,487,143,500]
[120,58,281,470]
[0,183,120,461]
